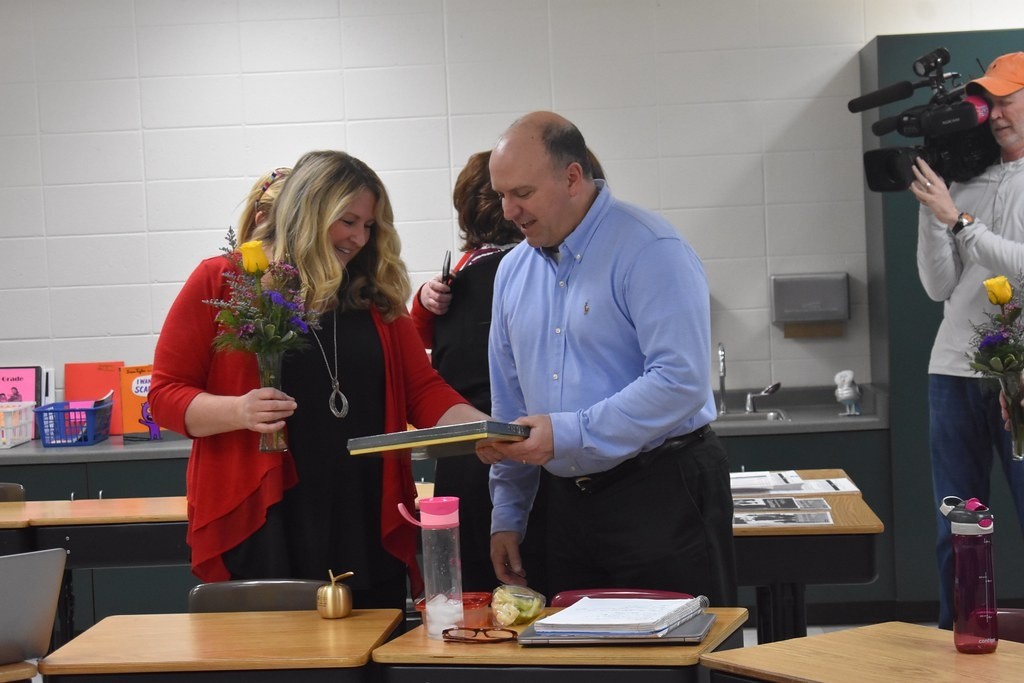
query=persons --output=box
[911,51,1024,632]
[147,150,505,628]
[411,153,529,596]
[486,110,742,603]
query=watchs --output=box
[951,211,974,235]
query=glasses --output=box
[254,167,294,215]
[442,626,518,644]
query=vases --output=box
[998,368,1024,461]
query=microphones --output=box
[847,72,958,113]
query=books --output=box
[66,360,173,439]
[534,591,709,637]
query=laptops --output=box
[517,613,714,644]
[0,548,67,666]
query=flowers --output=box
[963,265,1024,457]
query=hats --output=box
[966,51,1024,98]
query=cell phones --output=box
[441,250,451,295]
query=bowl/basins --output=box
[416,591,490,631]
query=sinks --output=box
[711,412,783,422]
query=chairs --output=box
[189,579,330,613]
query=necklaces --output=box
[308,302,349,418]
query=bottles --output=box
[939,496,998,653]
[397,496,465,640]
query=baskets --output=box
[0,402,38,449]
[31,400,115,447]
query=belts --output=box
[554,424,713,499]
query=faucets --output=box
[717,343,730,416]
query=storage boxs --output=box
[33,399,114,447]
[0,401,36,449]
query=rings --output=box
[494,461,497,463]
[523,459,526,465]
[926,181,931,188]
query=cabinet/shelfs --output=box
[0,430,205,632]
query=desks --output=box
[0,496,192,571]
[38,608,402,683]
[731,495,884,645]
[729,468,863,645]
[372,606,749,683]
[700,620,1024,683]
